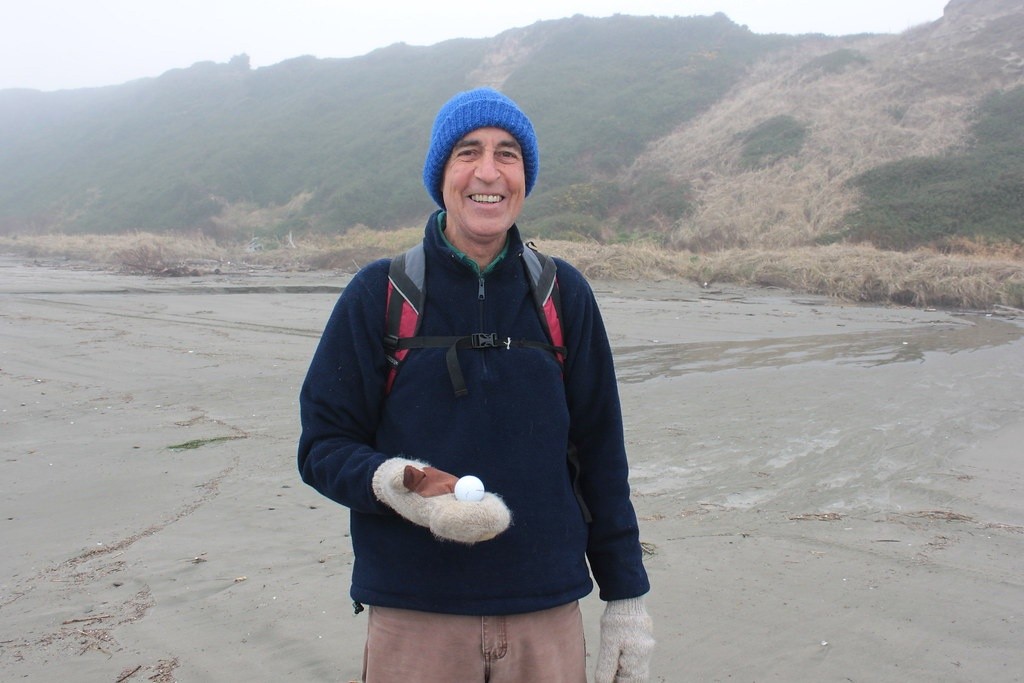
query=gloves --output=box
[594,596,655,683]
[372,457,511,543]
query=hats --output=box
[422,87,538,212]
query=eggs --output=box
[454,476,485,502]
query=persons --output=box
[297,89,653,682]
